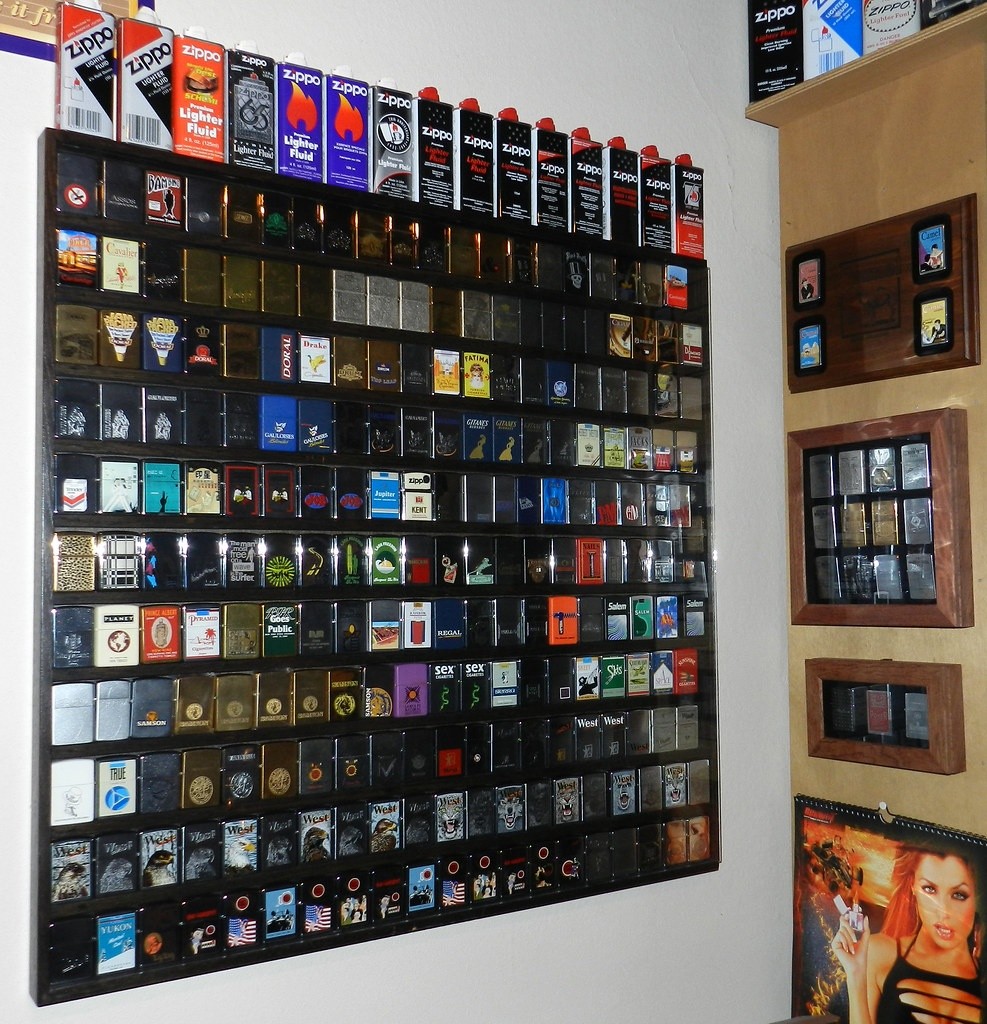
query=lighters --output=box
[916,225,947,275]
[796,259,820,303]
[833,890,865,933]
[797,324,822,370]
[809,445,936,603]
[836,688,930,741]
[921,299,949,347]
[41,150,717,982]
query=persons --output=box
[830,835,987,1024]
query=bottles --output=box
[414,89,455,206]
[368,79,418,200]
[601,136,640,245]
[494,107,534,220]
[801,0,862,81]
[640,144,669,252]
[117,0,175,150]
[275,51,322,179]
[321,66,370,189]
[223,41,276,169]
[671,153,704,256]
[54,0,116,140]
[175,23,225,157]
[531,118,567,232]
[453,98,494,213]
[747,0,803,100]
[566,126,604,239]
[863,0,921,53]
